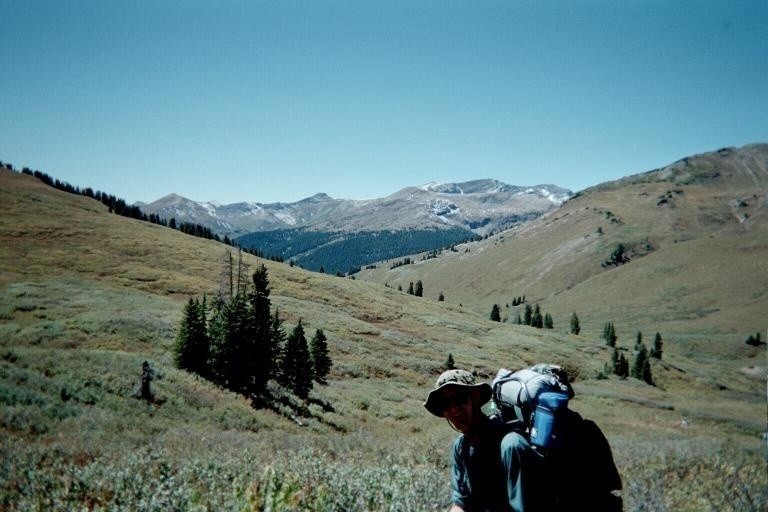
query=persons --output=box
[423,370,547,512]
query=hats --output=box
[422,368,492,417]
